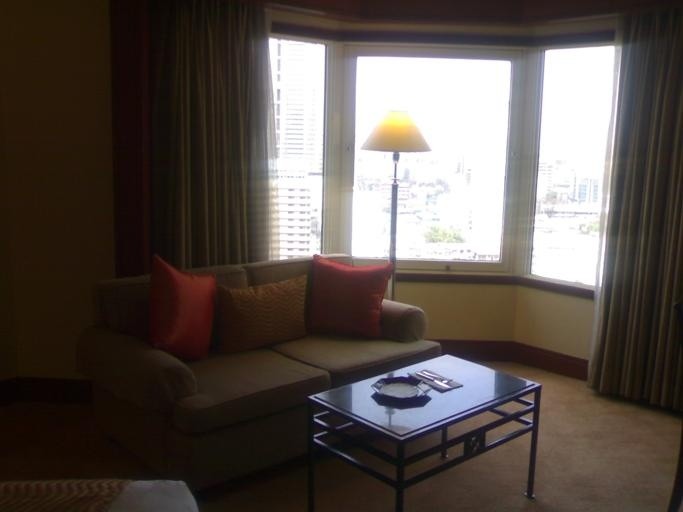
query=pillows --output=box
[141,253,394,361]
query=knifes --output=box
[416,371,452,389]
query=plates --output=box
[371,376,432,399]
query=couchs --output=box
[76,254,444,494]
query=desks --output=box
[0,480,199,512]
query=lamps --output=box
[360,114,432,301]
